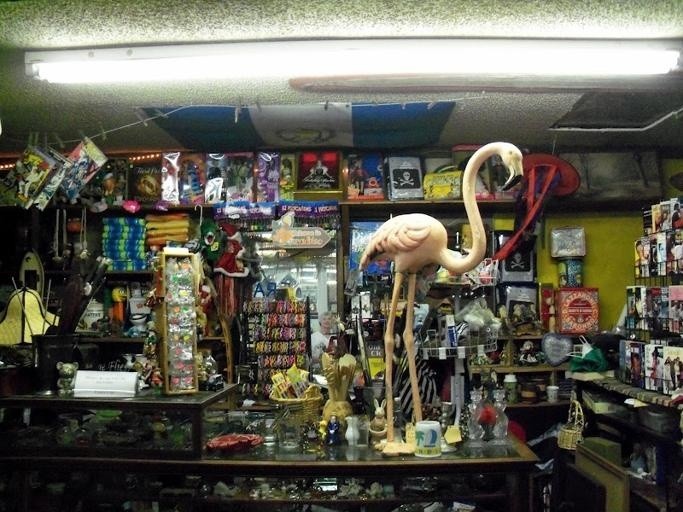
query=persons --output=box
[309,312,335,375]
[327,414,340,446]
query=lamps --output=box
[26,38,680,82]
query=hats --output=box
[0,288,60,348]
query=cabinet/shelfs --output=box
[0,148,65,380]
[0,384,242,460]
[620,199,683,385]
[66,150,573,408]
[556,373,683,512]
[0,427,538,511]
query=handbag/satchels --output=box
[566,336,609,373]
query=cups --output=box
[414,422,441,457]
[547,386,559,403]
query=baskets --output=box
[269,385,323,426]
[558,400,584,450]
